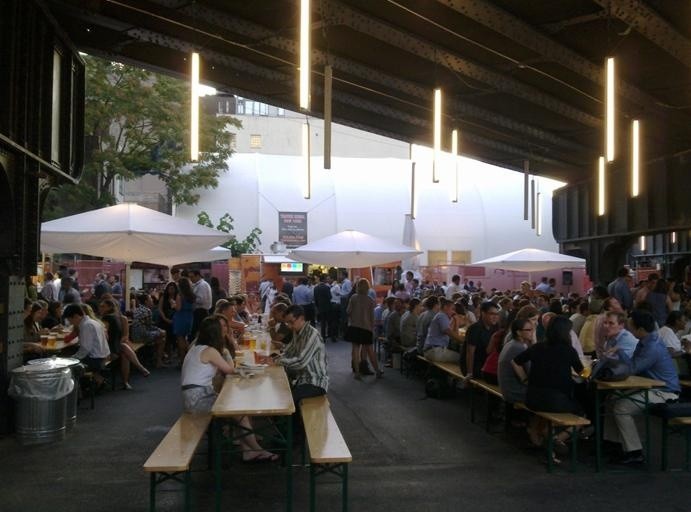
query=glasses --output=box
[285,316,299,326]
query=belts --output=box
[423,345,440,353]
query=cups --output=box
[47,336,56,348]
[40,335,48,346]
[243,334,267,364]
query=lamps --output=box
[532,180,534,228]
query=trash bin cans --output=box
[28,356,80,429]
[11,363,73,446]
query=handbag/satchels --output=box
[592,359,630,381]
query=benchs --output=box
[377,335,591,472]
[649,411,691,471]
[679,378,690,385]
[298,395,353,512]
[142,413,213,511]
[81,340,144,409]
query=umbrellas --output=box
[138,245,232,279]
[284,229,425,281]
[40,201,236,310]
[470,247,588,289]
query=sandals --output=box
[552,437,568,449]
[543,456,561,464]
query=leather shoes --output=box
[620,453,646,464]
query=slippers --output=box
[241,453,262,465]
[256,453,281,462]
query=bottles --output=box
[257,316,262,329]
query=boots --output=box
[352,359,384,379]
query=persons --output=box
[26,267,227,391]
[373,264,691,467]
[348,276,384,381]
[179,293,327,463]
[258,268,351,342]
